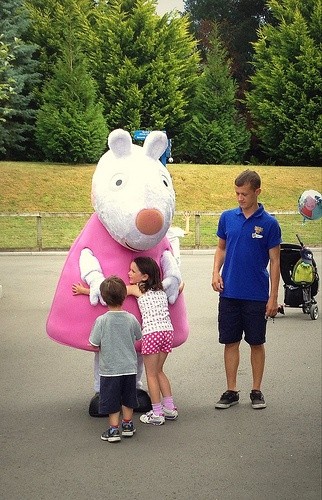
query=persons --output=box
[89,275,142,441]
[72,256,184,425]
[212,169,282,408]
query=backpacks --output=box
[292,258,315,285]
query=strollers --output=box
[277,234,320,320]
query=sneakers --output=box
[250,390,266,408]
[140,410,165,425]
[101,426,121,441]
[162,407,178,419]
[121,419,136,436]
[215,390,239,408]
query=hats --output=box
[302,250,313,266]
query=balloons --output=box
[298,190,322,220]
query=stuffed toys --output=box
[47,129,189,418]
